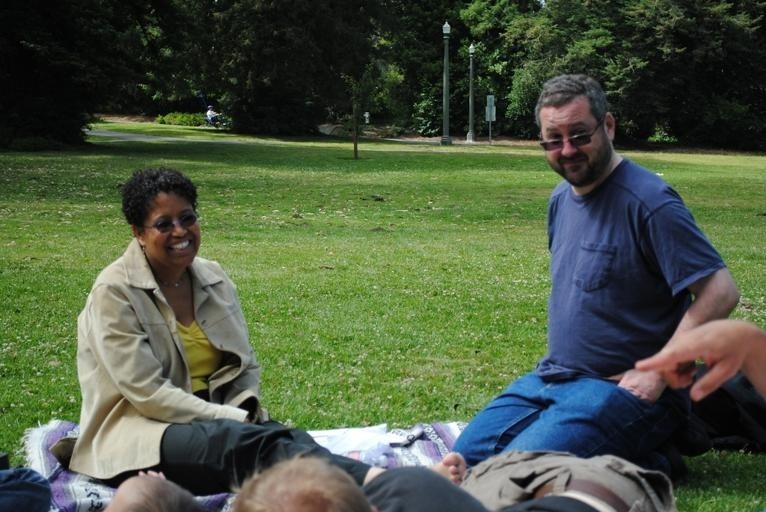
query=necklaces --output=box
[151,273,193,292]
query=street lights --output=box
[466,43,477,143]
[440,21,451,146]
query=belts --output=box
[533,475,630,511]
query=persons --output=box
[97,469,205,511]
[630,316,765,403]
[206,104,227,126]
[450,71,742,489]
[65,163,462,500]
[223,449,678,512]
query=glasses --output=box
[143,213,201,232]
[538,120,604,152]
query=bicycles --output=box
[204,113,233,127]
